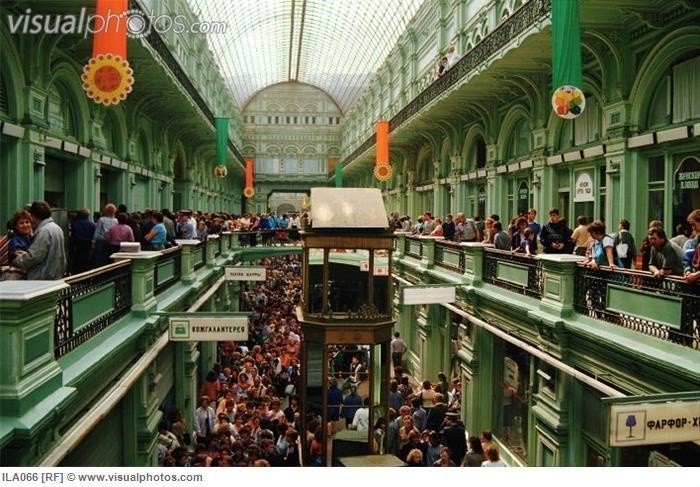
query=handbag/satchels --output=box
[616,232,629,259]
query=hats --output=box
[447,412,458,420]
